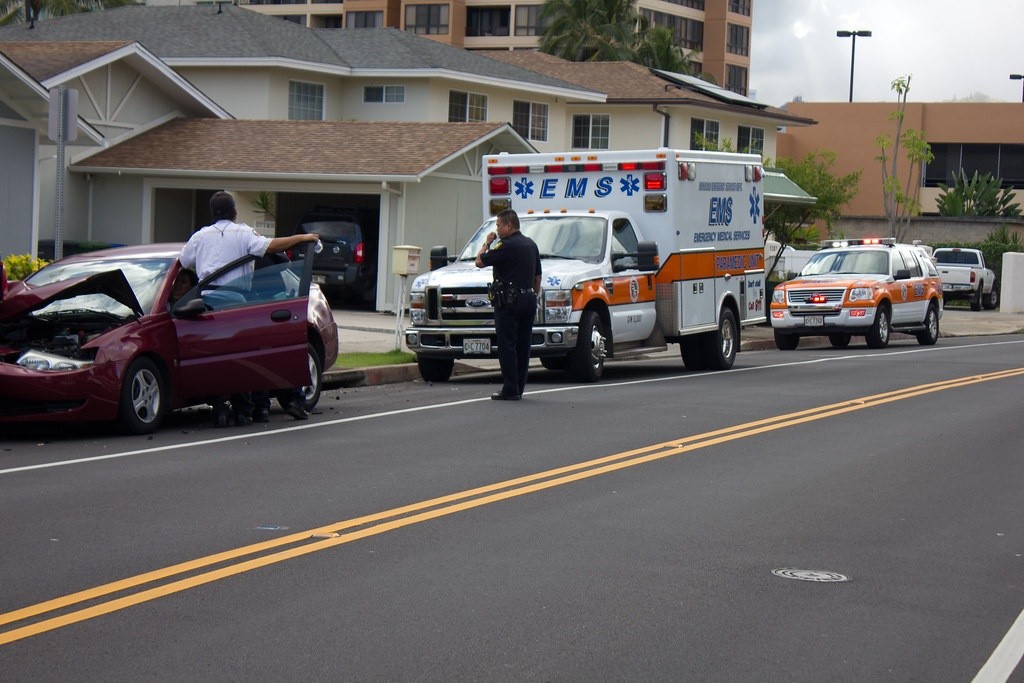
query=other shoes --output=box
[252,404,270,422]
[233,412,254,425]
[286,399,310,420]
[216,411,230,429]
[491,389,521,401]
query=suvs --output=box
[770,238,944,350]
[286,209,379,308]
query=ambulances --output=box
[404,146,766,387]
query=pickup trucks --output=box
[932,246,999,312]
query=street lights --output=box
[836,31,872,103]
[1010,74,1024,102]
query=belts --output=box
[515,287,535,294]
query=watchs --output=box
[482,243,490,249]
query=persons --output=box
[177,192,318,428]
[476,210,542,399]
[166,270,199,313]
[252,253,309,423]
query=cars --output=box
[1,239,339,436]
